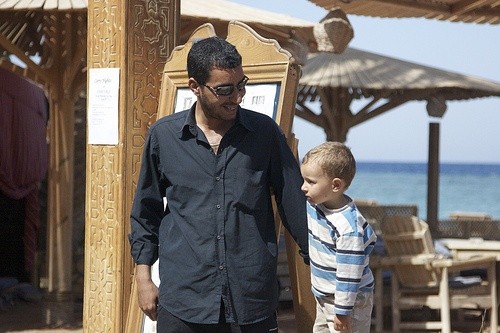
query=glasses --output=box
[202,75,250,96]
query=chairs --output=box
[381,215,500,332]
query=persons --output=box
[128,35,308,333]
[300,142,377,333]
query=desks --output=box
[435,238,500,277]
